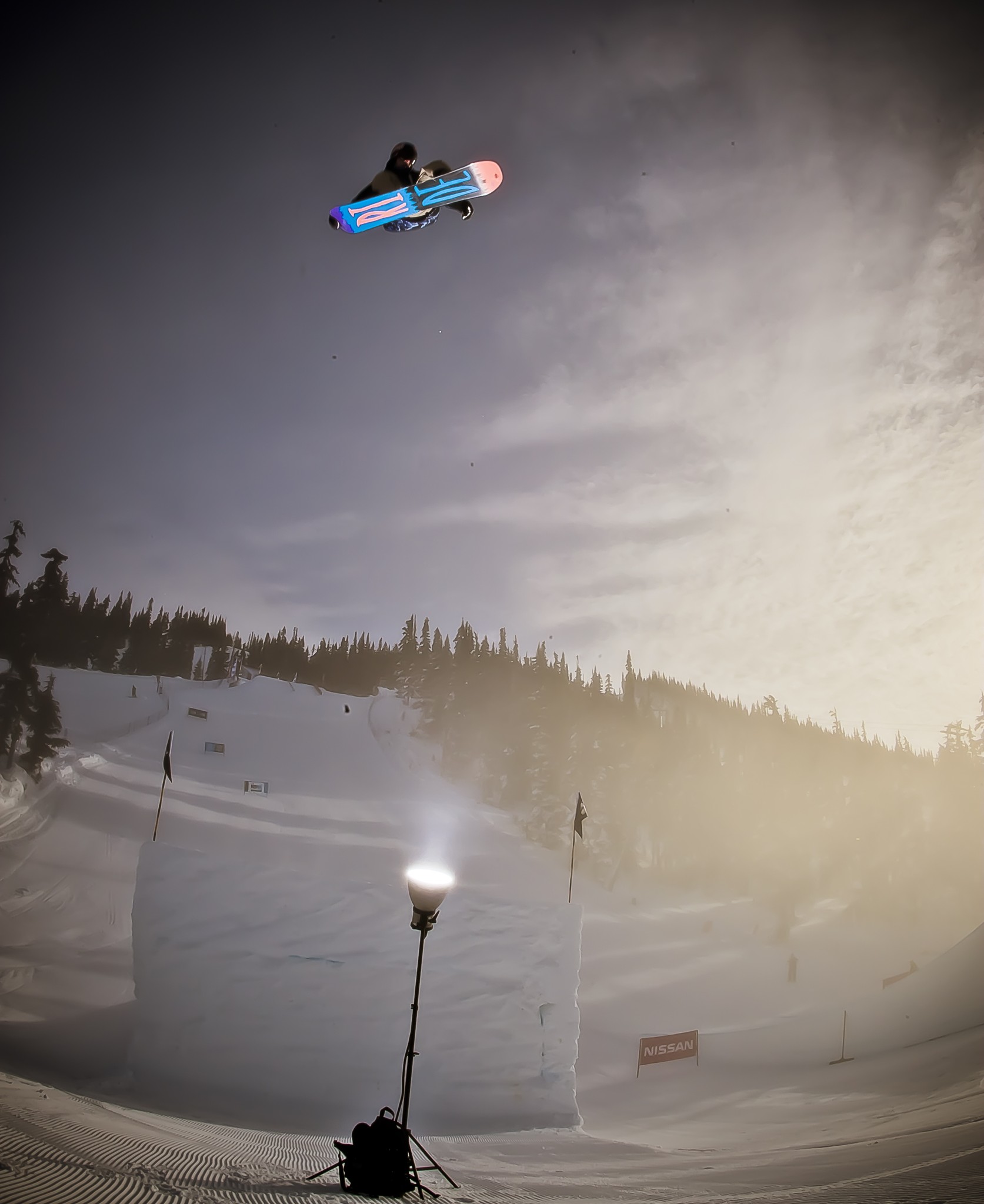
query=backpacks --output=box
[359,1111,411,1193]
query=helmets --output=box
[389,143,418,165]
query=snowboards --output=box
[328,160,504,236]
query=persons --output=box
[345,705,350,713]
[328,141,475,234]
